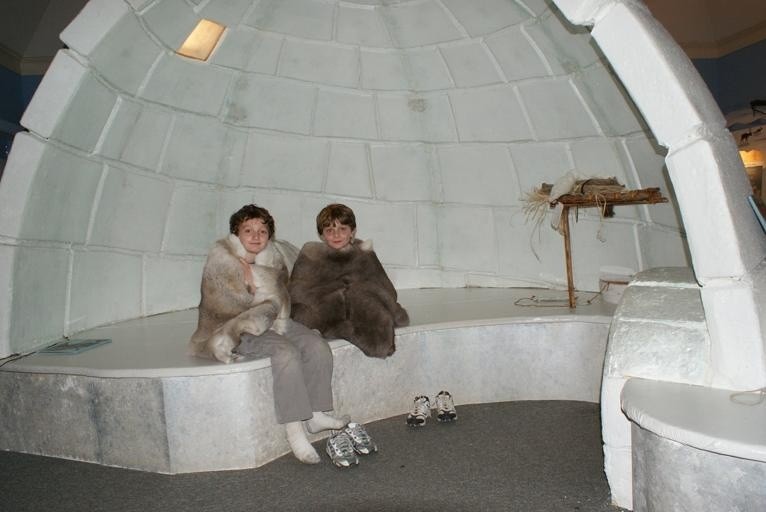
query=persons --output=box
[287,204,410,360]
[184,204,351,467]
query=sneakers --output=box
[436,390,457,423]
[406,396,431,427]
[344,423,378,456]
[326,432,360,470]
